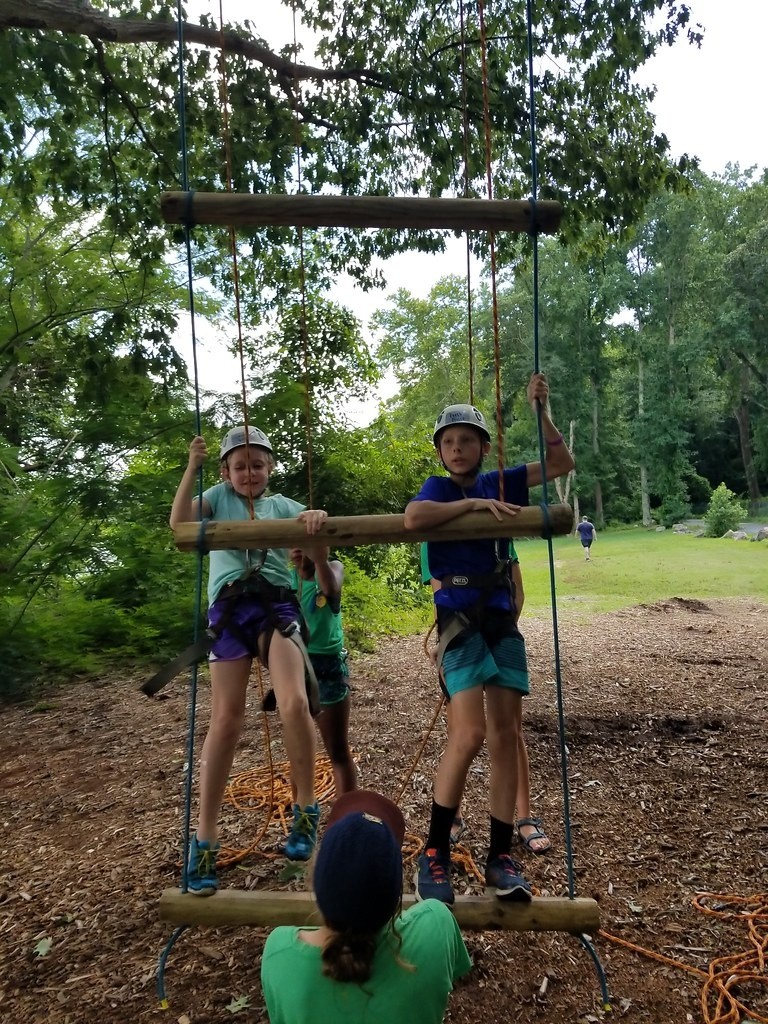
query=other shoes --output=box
[585,557,590,562]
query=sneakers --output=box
[286,802,320,861]
[413,848,455,907]
[484,854,533,901]
[187,832,219,895]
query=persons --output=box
[404,372,575,908]
[575,516,596,561]
[170,426,355,897]
[261,790,472,1024]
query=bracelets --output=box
[545,433,563,445]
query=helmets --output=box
[219,426,273,460]
[433,403,491,447]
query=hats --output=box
[313,789,406,934]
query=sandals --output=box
[449,820,468,847]
[516,818,551,853]
